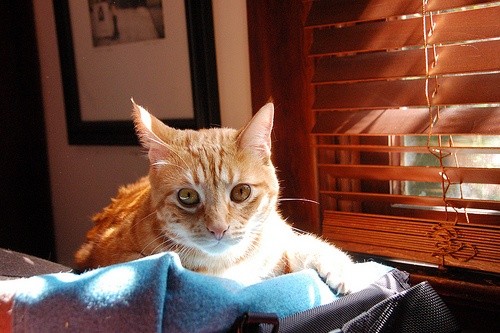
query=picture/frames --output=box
[49,1,222,146]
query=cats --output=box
[73,97,363,297]
